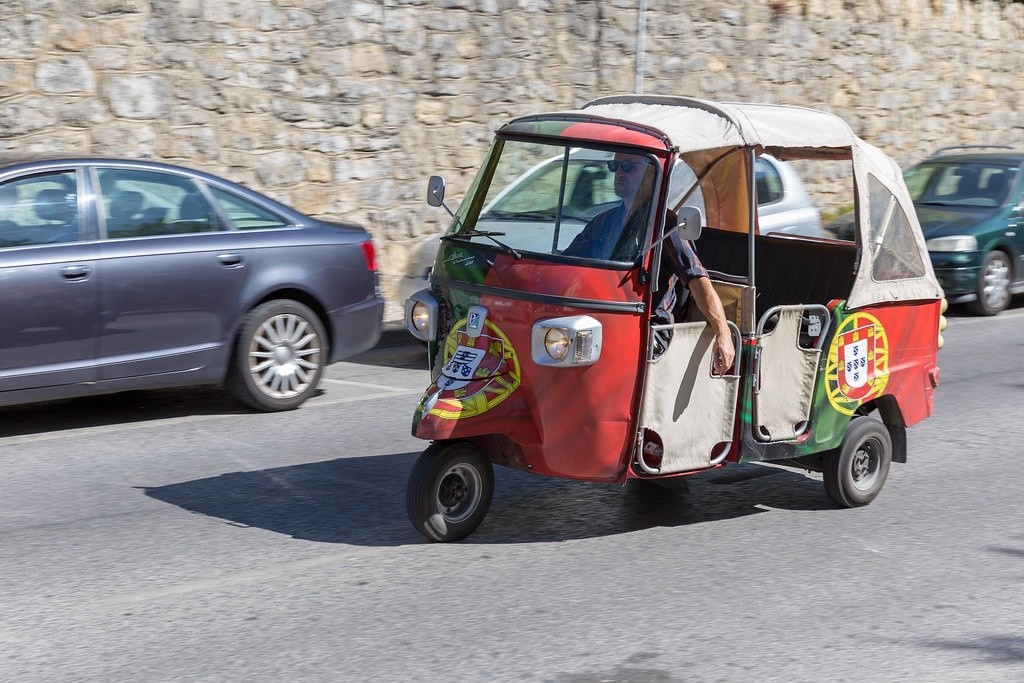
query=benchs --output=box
[695,224,861,344]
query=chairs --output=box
[957,173,1007,200]
[0,186,206,247]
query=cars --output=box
[823,144,1024,315]
[397,148,824,342]
[0,158,384,411]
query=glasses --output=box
[607,159,652,172]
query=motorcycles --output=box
[405,94,949,543]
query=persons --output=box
[557,150,735,379]
